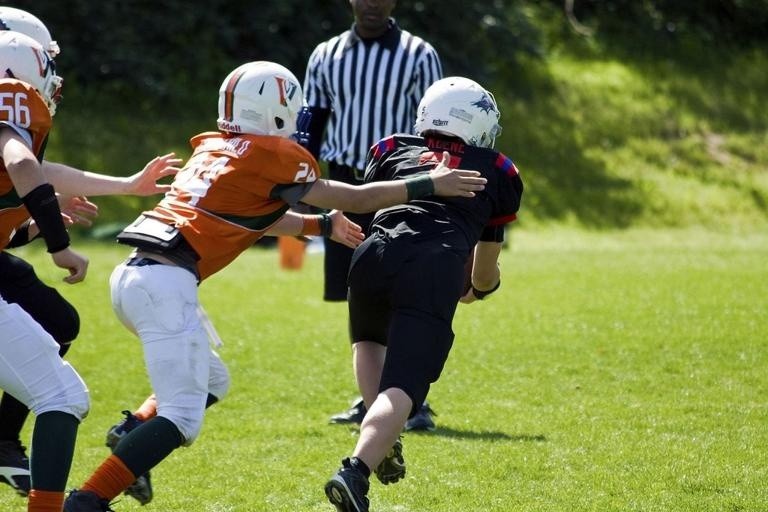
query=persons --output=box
[296,1,439,433]
[324,77,523,512]
[0,33,91,512]
[62,62,488,512]
[0,19,184,496]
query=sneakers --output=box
[328,398,366,428]
[373,435,407,486]
[63,488,122,512]
[0,440,30,493]
[402,399,441,434]
[104,409,156,506]
[322,457,373,511]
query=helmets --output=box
[216,61,314,142]
[0,5,62,62]
[0,30,63,118]
[413,76,504,150]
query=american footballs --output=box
[461,249,473,297]
